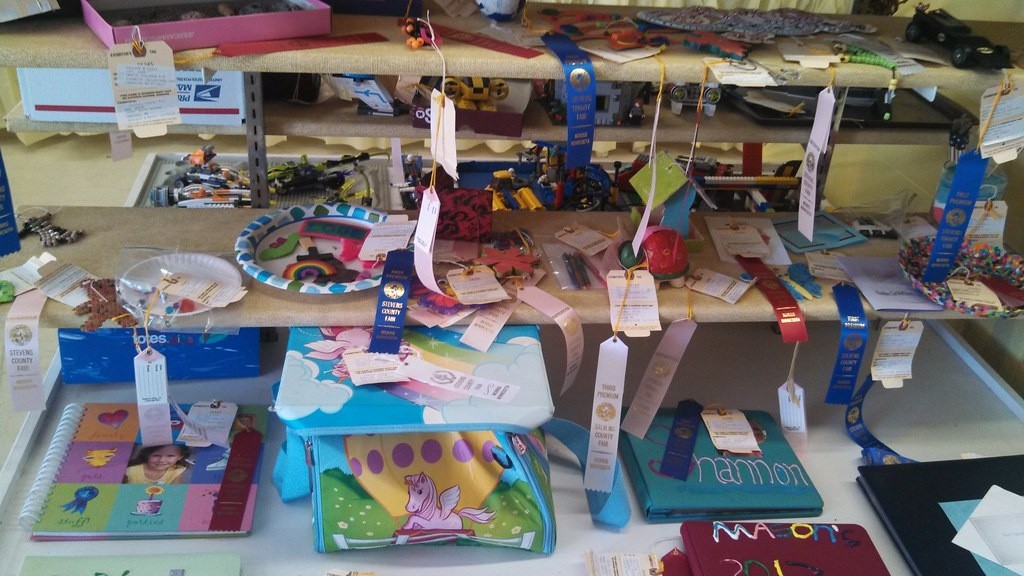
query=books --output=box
[856,453,1024,576]
[16,401,272,542]
[619,407,825,524]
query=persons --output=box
[121,444,190,485]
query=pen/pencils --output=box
[561,252,606,288]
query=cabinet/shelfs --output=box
[0,0,1024,328]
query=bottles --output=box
[235,200,402,293]
[121,251,243,316]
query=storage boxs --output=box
[58,327,260,385]
[0,0,536,137]
